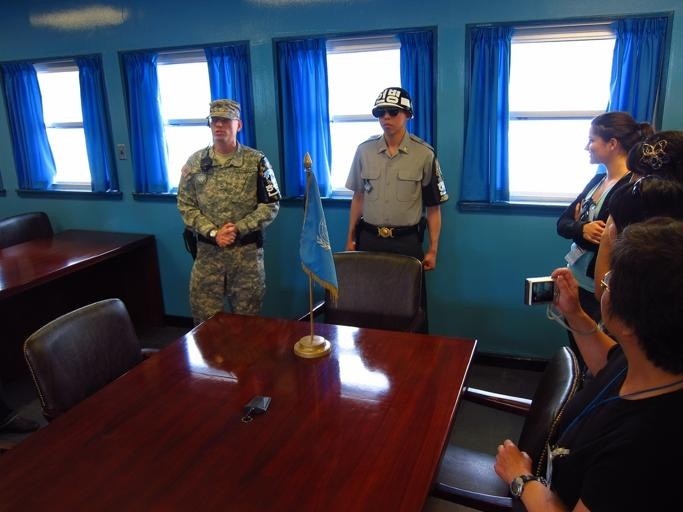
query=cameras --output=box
[525,276,559,305]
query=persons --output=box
[345,87,449,270]
[557,113,683,374]
[176,99,282,326]
[494,217,683,512]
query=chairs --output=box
[25,299,160,423]
[295,252,427,335]
[1,211,53,249]
[429,344,583,512]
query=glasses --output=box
[372,107,402,118]
[600,270,613,293]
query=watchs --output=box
[209,228,220,243]
[509,474,536,499]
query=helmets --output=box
[372,87,415,120]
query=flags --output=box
[300,168,339,308]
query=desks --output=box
[0,229,166,350]
[0,311,479,512]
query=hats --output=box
[209,100,241,120]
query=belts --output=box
[199,233,255,248]
[363,221,419,238]
